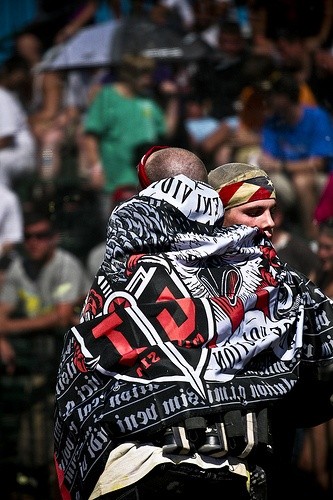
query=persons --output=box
[1,0,333,372]
[50,144,333,500]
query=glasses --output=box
[24,228,54,240]
